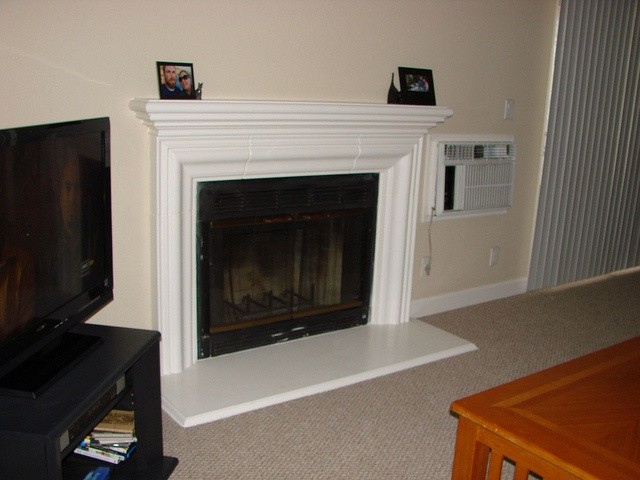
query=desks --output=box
[449,336,640,480]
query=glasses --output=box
[178,75,189,81]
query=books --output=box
[73,409,138,465]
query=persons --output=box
[177,70,193,97]
[161,65,180,97]
[418,76,426,92]
[422,78,429,92]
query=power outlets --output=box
[420,256,433,278]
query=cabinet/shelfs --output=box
[3,324,163,480]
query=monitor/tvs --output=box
[0,116,114,399]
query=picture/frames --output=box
[155,60,198,100]
[398,66,438,106]
